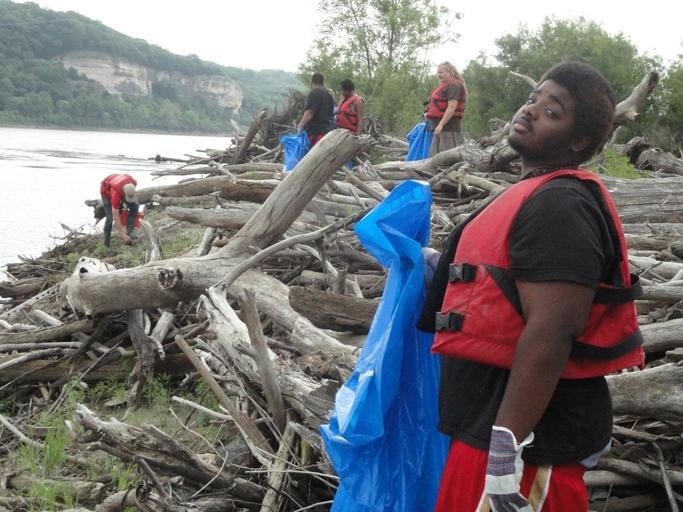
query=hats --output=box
[124,183,137,202]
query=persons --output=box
[100,172,139,255]
[422,61,468,159]
[297,72,365,169]
[422,60,644,512]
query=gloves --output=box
[475,425,535,512]
[422,247,442,271]
